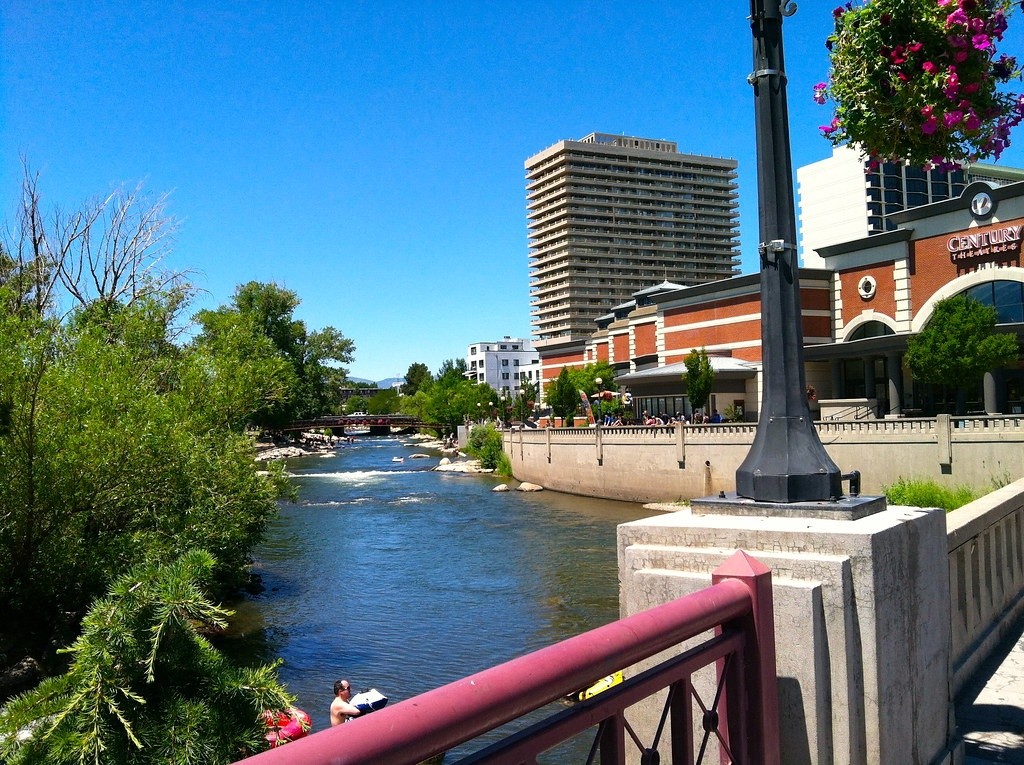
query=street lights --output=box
[519,389,525,428]
[501,396,506,428]
[477,403,481,424]
[596,378,603,425]
[489,402,493,422]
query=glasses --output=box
[342,686,350,691]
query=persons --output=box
[613,415,628,426]
[642,410,691,425]
[329,679,360,727]
[603,415,614,426]
[703,413,709,424]
[704,408,720,423]
[700,414,703,424]
[694,410,700,424]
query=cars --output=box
[354,412,366,416]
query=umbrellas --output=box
[591,389,622,398]
